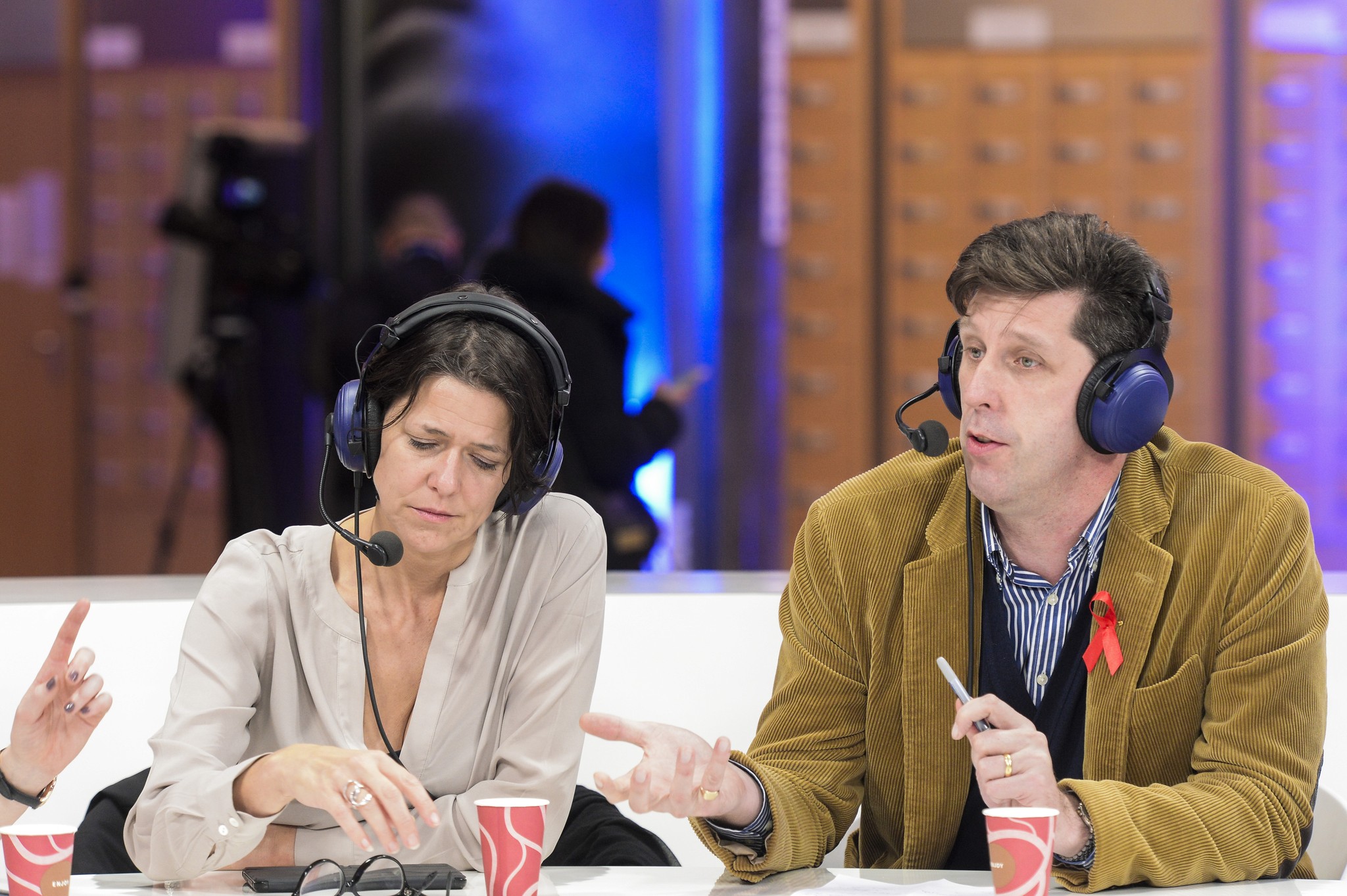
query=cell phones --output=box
[242,864,465,891]
[679,360,713,387]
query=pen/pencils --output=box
[935,656,990,732]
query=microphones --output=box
[893,384,950,458]
[317,414,403,567]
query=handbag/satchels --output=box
[589,485,658,561]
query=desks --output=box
[0,861,1344,896]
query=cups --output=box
[473,798,550,896]
[0,824,77,896]
[982,807,1061,895]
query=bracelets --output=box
[1056,784,1095,863]
[0,747,55,811]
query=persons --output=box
[0,596,112,832]
[319,174,711,571]
[578,212,1327,896]
[121,283,610,882]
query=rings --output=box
[698,784,721,802]
[342,778,373,809]
[1003,753,1014,777]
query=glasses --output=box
[291,854,453,896]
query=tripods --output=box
[144,311,285,577]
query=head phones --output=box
[331,285,572,516]
[940,263,1175,455]
